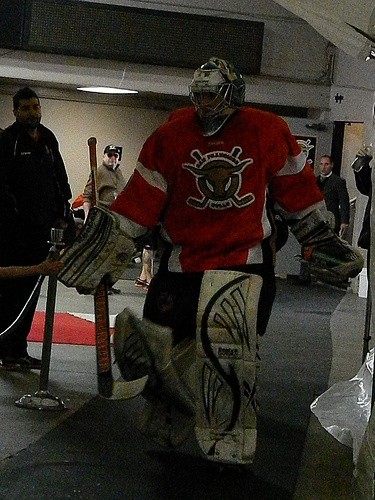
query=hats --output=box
[103,144,120,154]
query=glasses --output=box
[107,152,118,158]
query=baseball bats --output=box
[86,136,151,400]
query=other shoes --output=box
[134,277,150,291]
[107,288,121,295]
[0,357,32,372]
[23,355,42,369]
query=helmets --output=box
[187,55,246,137]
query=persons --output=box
[83,144,126,295]
[317,154,350,238]
[0,87,71,369]
[55,56,364,464]
[134,245,154,292]
[353,145,373,284]
